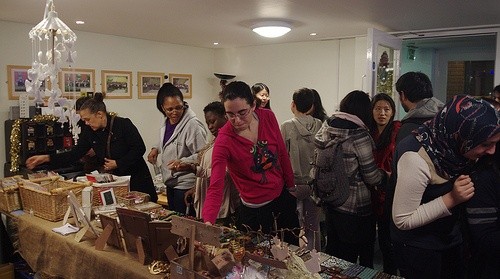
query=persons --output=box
[370,92,401,275]
[25,92,159,204]
[202,81,300,247]
[279,87,329,251]
[168,101,240,225]
[385,85,500,279]
[74,96,97,168]
[315,90,389,270]
[251,82,271,110]
[147,82,209,217]
[391,72,445,144]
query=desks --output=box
[0,176,168,279]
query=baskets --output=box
[0,174,129,222]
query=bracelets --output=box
[151,147,156,149]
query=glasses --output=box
[256,95,271,100]
[225,104,253,120]
[162,104,184,113]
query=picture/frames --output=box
[57,67,96,100]
[137,71,165,99]
[7,64,52,102]
[169,73,193,98]
[101,69,134,99]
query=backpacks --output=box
[313,134,361,207]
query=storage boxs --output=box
[82,186,93,220]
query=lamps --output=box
[249,21,293,39]
[214,73,236,87]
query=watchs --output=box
[288,185,297,192]
